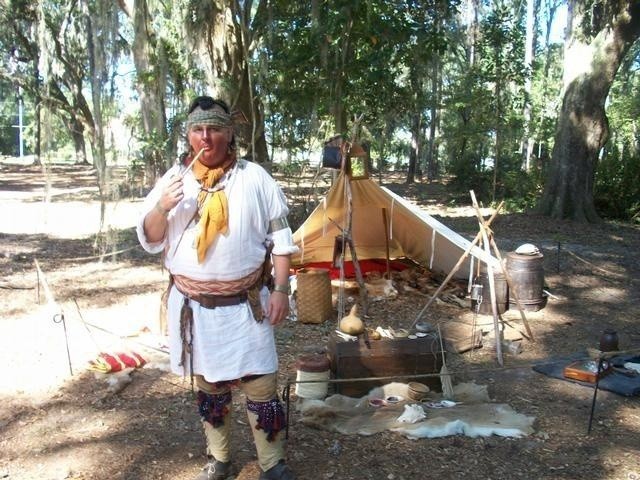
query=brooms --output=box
[437,322,454,399]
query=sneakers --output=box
[194,454,237,480]
[259,460,297,480]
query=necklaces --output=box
[196,164,234,192]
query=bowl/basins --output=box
[415,321,432,332]
[407,381,429,399]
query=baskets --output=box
[296,267,332,325]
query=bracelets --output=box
[156,200,170,217]
[274,285,289,293]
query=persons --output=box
[135,95,300,479]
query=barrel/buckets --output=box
[507,250,544,312]
[473,273,507,315]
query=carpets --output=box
[297,382,535,441]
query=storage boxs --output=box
[326,328,448,398]
